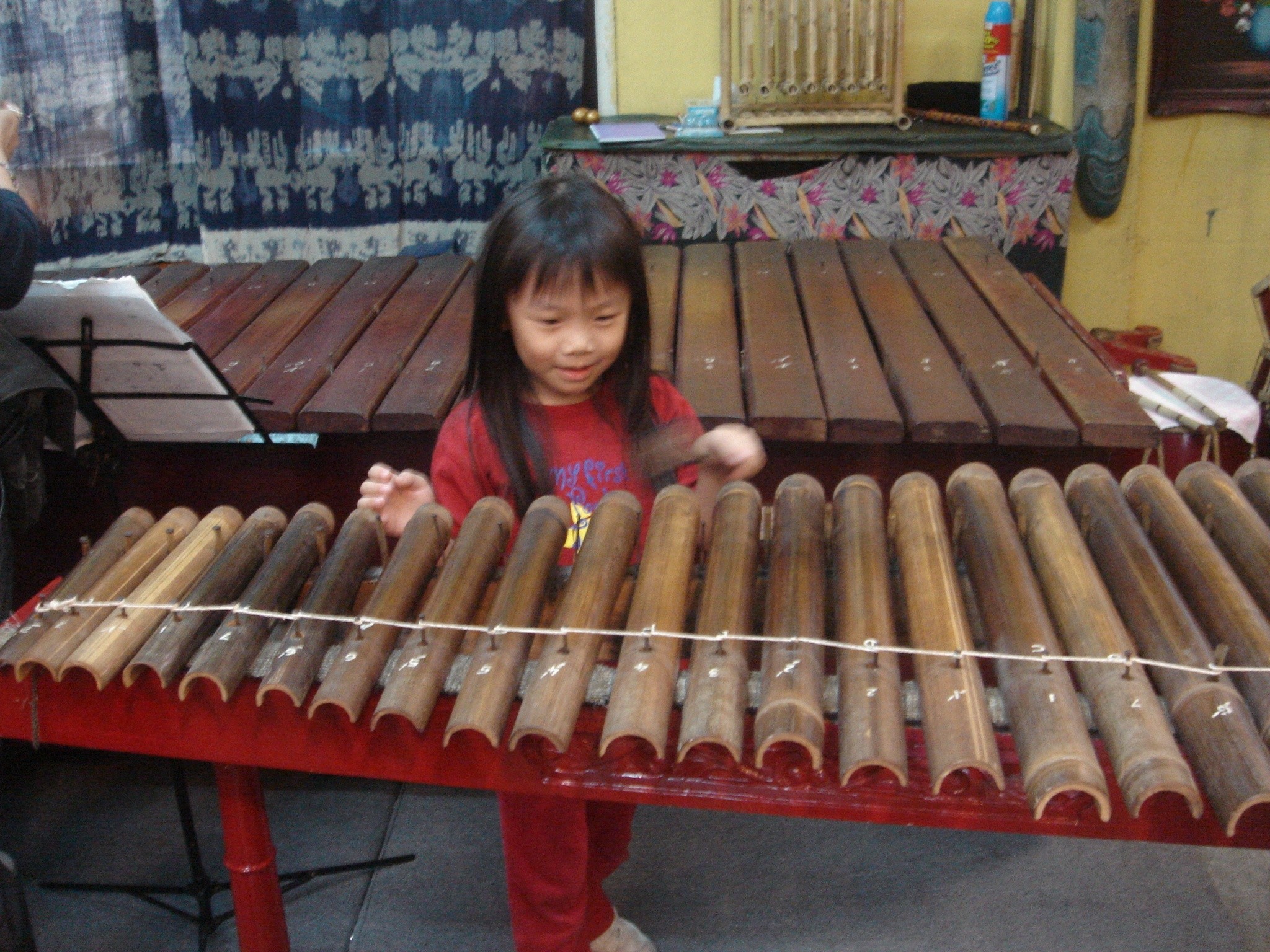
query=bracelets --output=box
[0,162,20,193]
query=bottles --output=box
[980,1,1013,122]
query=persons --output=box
[357,177,767,952]
[0,98,39,311]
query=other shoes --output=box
[591,906,655,952]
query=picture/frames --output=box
[1146,0,1270,118]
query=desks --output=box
[540,110,1077,299]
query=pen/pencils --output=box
[659,124,678,131]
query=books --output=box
[589,123,665,143]
